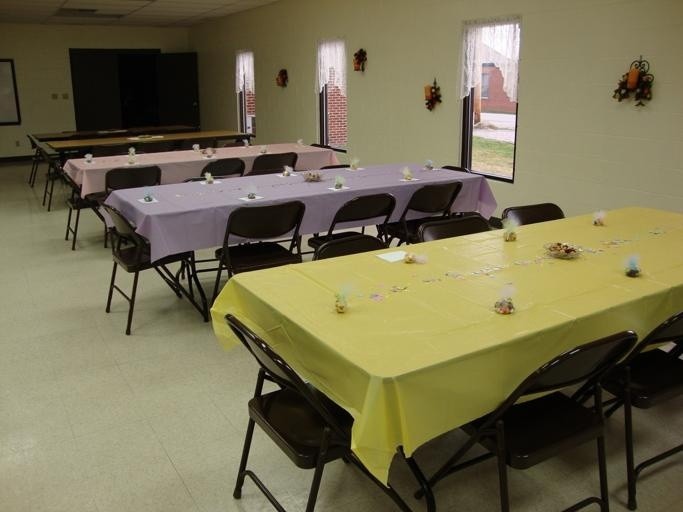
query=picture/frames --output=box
[0,58,20,125]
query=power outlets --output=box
[63,93,68,99]
[51,93,58,100]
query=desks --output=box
[61,142,341,214]
[47,131,256,156]
[211,209,683,512]
[27,124,202,144]
[105,163,496,324]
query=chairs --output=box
[570,307,683,510]
[52,163,111,252]
[37,143,78,212]
[310,144,332,149]
[182,176,225,182]
[414,328,637,512]
[309,192,399,260]
[244,152,299,175]
[88,194,211,335]
[77,144,115,159]
[442,165,472,175]
[416,212,492,245]
[201,158,247,180]
[221,312,413,512]
[318,165,352,170]
[211,200,305,307]
[311,233,389,262]
[376,181,464,248]
[502,203,564,229]
[105,166,163,194]
[23,133,65,187]
